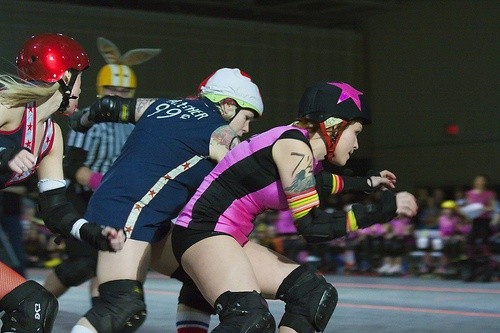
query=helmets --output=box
[17,33,91,83]
[298,80,371,123]
[198,67,264,116]
[96,63,138,98]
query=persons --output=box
[251,176,500,282]
[0,33,127,333]
[172,81,396,333]
[69,68,264,333]
[38,65,138,305]
